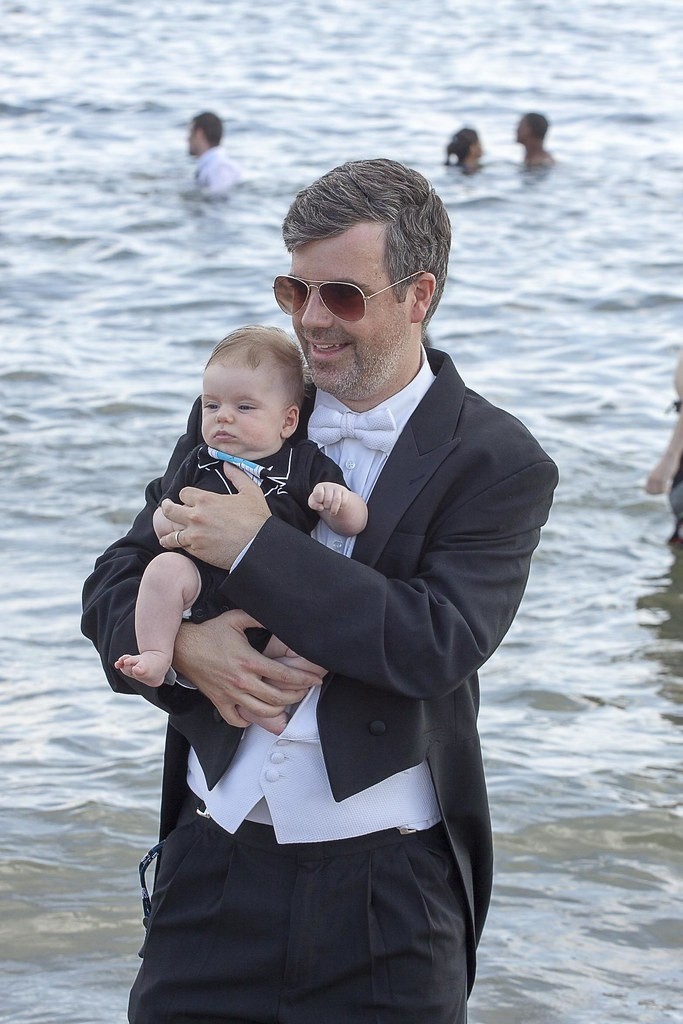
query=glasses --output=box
[272,269,427,322]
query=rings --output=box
[175,531,183,546]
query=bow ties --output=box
[306,405,397,455]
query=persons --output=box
[645,352,683,547]
[81,160,558,1024]
[189,112,242,187]
[517,113,553,164]
[114,324,368,735]
[446,129,483,168]
